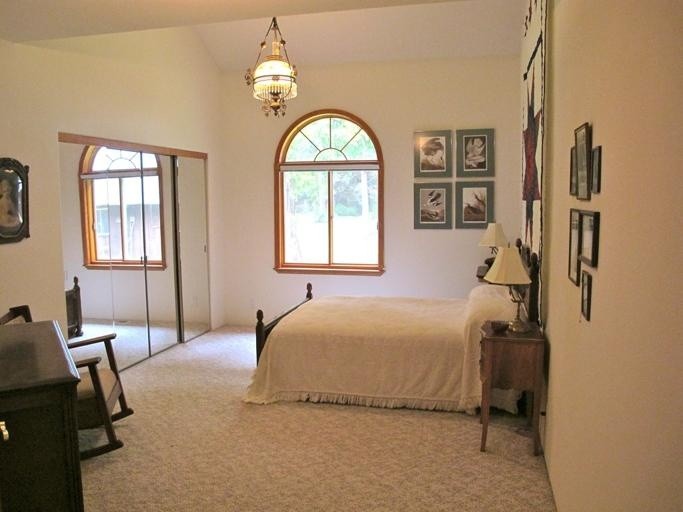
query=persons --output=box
[0,178,20,227]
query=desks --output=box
[474,264,491,282]
[477,320,546,456]
[0,317,86,512]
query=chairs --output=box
[0,304,136,462]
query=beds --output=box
[65,273,84,339]
[239,237,539,415]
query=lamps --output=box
[476,222,510,259]
[243,15,302,119]
[483,244,532,335]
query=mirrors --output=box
[55,131,212,375]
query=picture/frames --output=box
[454,180,495,230]
[412,181,453,230]
[454,126,496,180]
[412,128,454,179]
[0,155,32,247]
[564,120,603,322]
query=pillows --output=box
[463,282,527,335]
